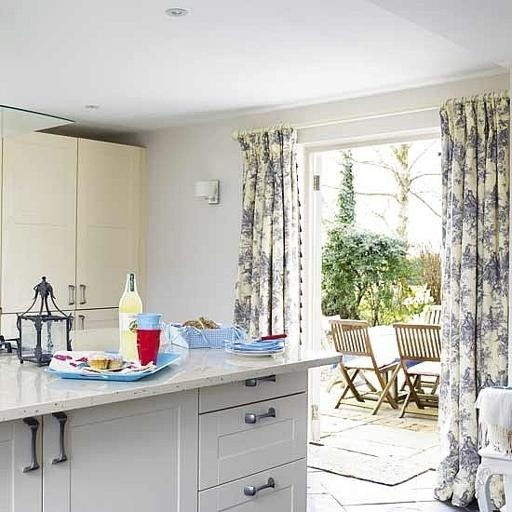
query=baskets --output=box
[169,322,235,349]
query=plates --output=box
[224,339,285,357]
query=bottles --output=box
[118,273,143,365]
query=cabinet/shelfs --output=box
[1,131,148,339]
[0,370,307,511]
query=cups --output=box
[136,314,162,366]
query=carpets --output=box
[306,413,438,486]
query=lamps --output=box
[193,179,220,205]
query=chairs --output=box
[328,305,443,418]
